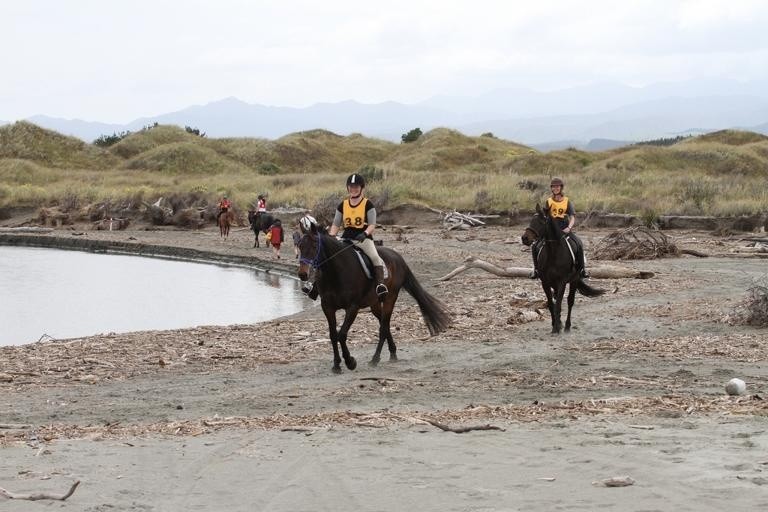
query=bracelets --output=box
[568,225,570,229]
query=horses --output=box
[216,205,234,237]
[248,210,273,248]
[522,203,608,338]
[297,221,457,374]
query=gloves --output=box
[355,231,366,243]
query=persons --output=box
[526,176,590,281]
[298,210,318,235]
[249,193,268,231]
[298,172,390,305]
[214,193,233,227]
[265,218,285,262]
[291,227,302,261]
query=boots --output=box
[301,281,319,301]
[577,246,590,278]
[215,218,219,227]
[529,247,539,279]
[250,219,256,231]
[373,264,388,301]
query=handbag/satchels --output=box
[266,225,273,241]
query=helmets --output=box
[257,194,264,200]
[550,177,563,190]
[223,195,228,199]
[346,173,365,191]
